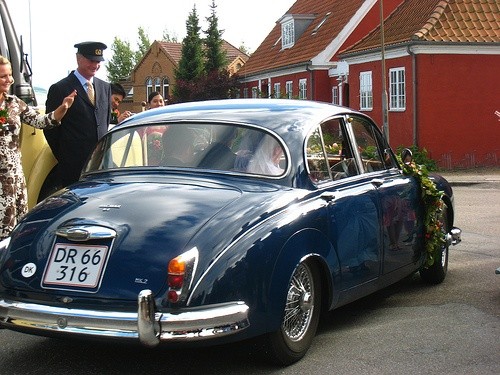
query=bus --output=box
[0,0,141,213]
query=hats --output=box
[74,41,107,61]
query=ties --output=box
[85,81,95,106]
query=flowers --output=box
[306,142,452,269]
[0,106,10,125]
[148,135,162,149]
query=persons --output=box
[42,42,113,177]
[385,196,416,251]
[136,91,168,139]
[0,55,77,243]
[159,125,285,175]
[109,82,132,123]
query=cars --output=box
[0,98,463,364]
